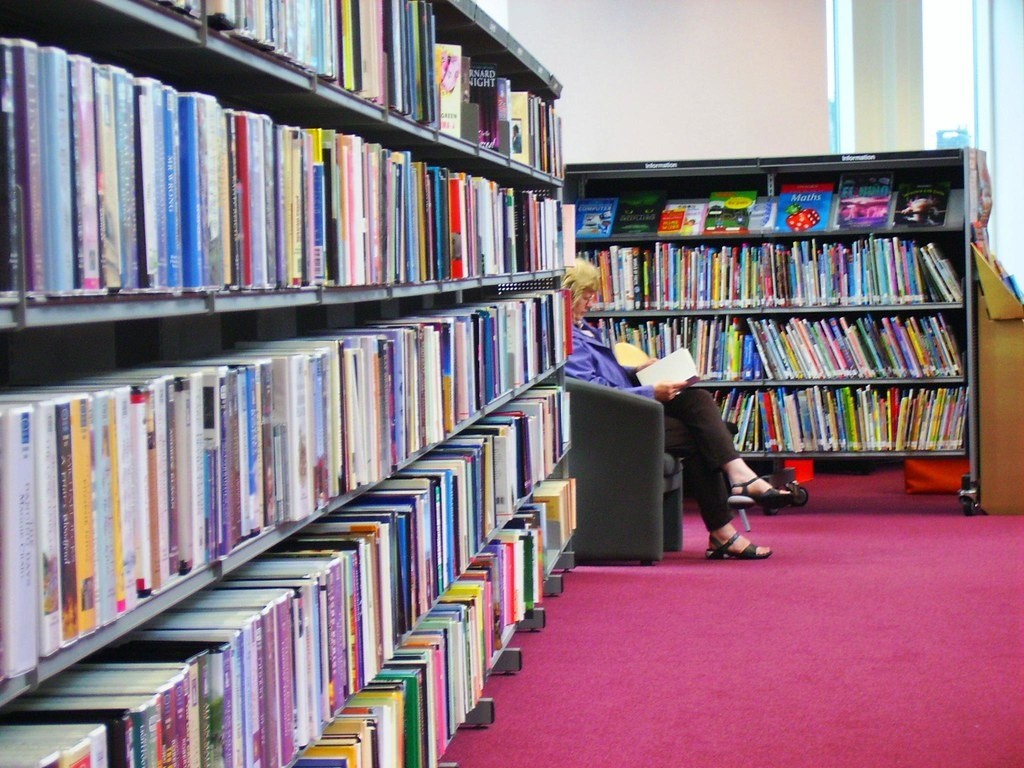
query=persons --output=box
[561,257,793,559]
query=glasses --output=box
[582,294,594,307]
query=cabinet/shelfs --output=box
[562,146,987,517]
[1,0,580,768]
[977,282,1024,516]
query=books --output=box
[583,312,968,383]
[2,39,577,305]
[705,386,973,453]
[0,286,564,688]
[1,378,576,768]
[133,0,571,178]
[970,216,1024,320]
[572,169,954,237]
[636,347,701,396]
[281,468,584,768]
[574,234,964,312]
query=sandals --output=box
[727,476,793,509]
[705,532,773,560]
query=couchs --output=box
[565,376,684,567]
